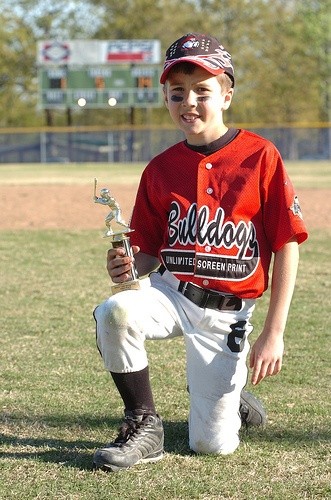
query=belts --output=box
[156,263,243,313]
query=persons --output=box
[92,31,309,471]
[94,188,130,237]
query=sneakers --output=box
[186,383,267,430]
[93,409,165,472]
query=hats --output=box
[159,33,235,89]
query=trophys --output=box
[93,179,141,296]
